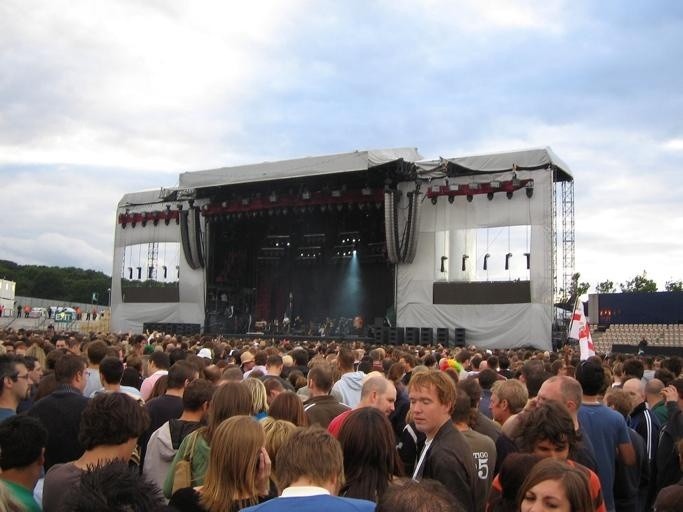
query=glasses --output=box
[11,374,30,379]
[79,372,90,377]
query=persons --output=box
[0,296,682,511]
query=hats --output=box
[196,348,212,360]
[240,352,255,367]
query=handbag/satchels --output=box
[171,460,191,495]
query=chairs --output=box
[586,322,683,356]
